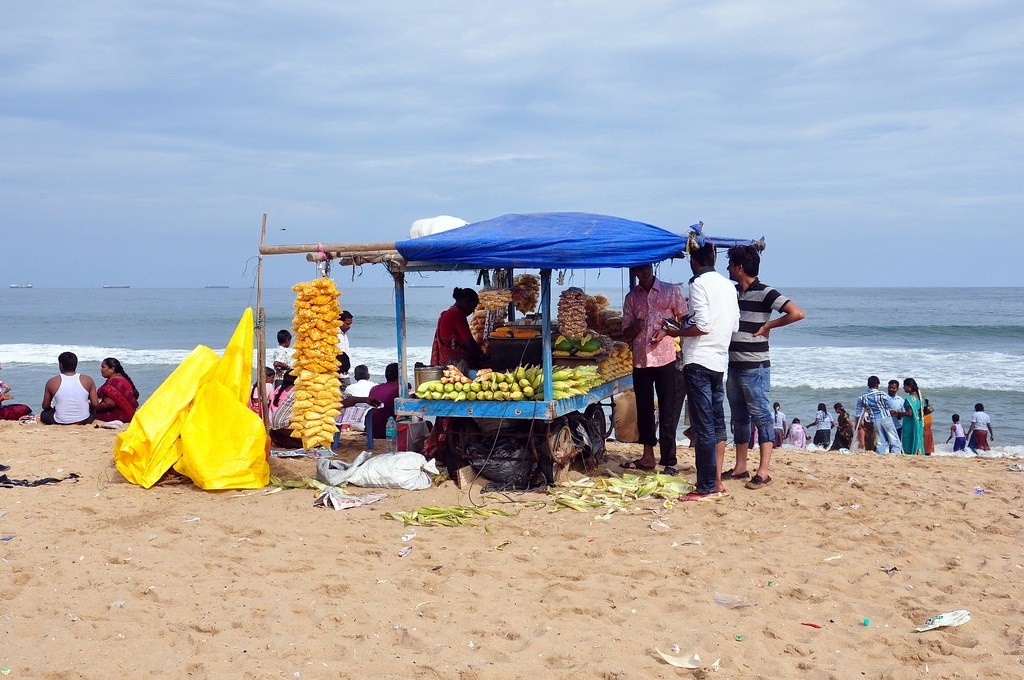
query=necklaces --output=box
[912,391,916,395]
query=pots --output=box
[487,334,543,370]
[414,362,447,393]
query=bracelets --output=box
[895,411,897,415]
[480,354,484,358]
[634,328,639,332]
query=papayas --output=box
[555,339,601,352]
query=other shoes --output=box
[746,473,773,489]
[722,469,749,481]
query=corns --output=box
[552,365,603,399]
[416,365,544,401]
[491,327,541,337]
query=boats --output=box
[205,285,229,288]
[10,281,32,288]
[103,281,129,288]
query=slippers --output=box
[660,467,680,477]
[679,491,709,502]
[618,459,656,470]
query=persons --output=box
[0,381,13,408]
[730,376,934,456]
[336,311,411,439]
[946,403,994,454]
[666,242,741,502]
[721,245,805,490]
[620,264,690,475]
[247,329,303,449]
[420,288,489,460]
[40,352,139,425]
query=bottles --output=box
[386,417,398,455]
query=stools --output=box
[331,408,374,450]
